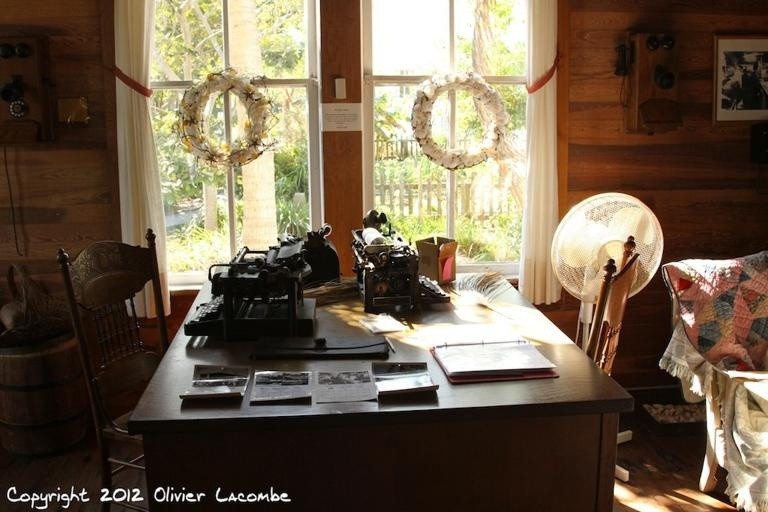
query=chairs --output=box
[58,227,178,510]
[570,233,639,375]
[660,252,768,511]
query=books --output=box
[430,340,560,384]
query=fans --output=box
[549,189,666,482]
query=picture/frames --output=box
[713,31,768,125]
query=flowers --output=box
[409,72,509,172]
[177,69,273,169]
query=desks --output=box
[128,271,634,510]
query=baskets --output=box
[0,264,71,346]
